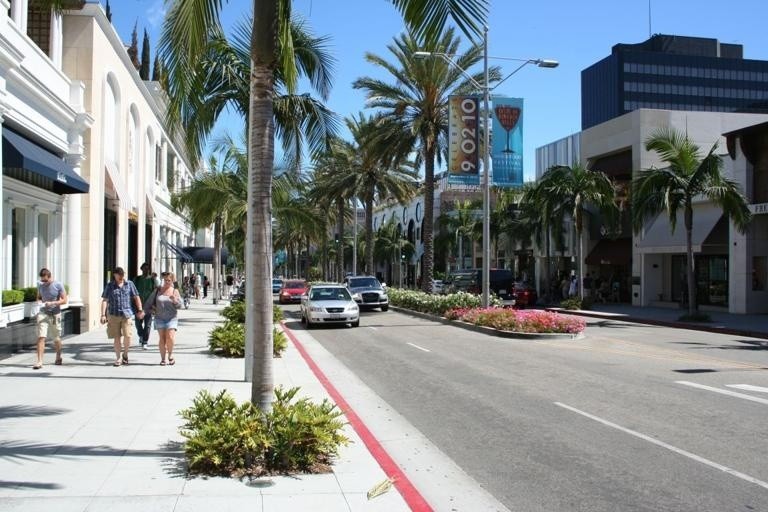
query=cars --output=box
[299,282,360,327]
[342,275,390,312]
[279,278,309,304]
[272,278,283,294]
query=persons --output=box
[100,262,210,367]
[226,272,234,300]
[559,270,631,305]
[32,268,67,369]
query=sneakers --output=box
[54,358,62,365]
[160,357,175,365]
[33,362,42,369]
[140,336,147,350]
[115,353,128,366]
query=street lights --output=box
[412,25,560,309]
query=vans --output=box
[434,268,515,300]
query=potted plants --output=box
[2,284,69,324]
